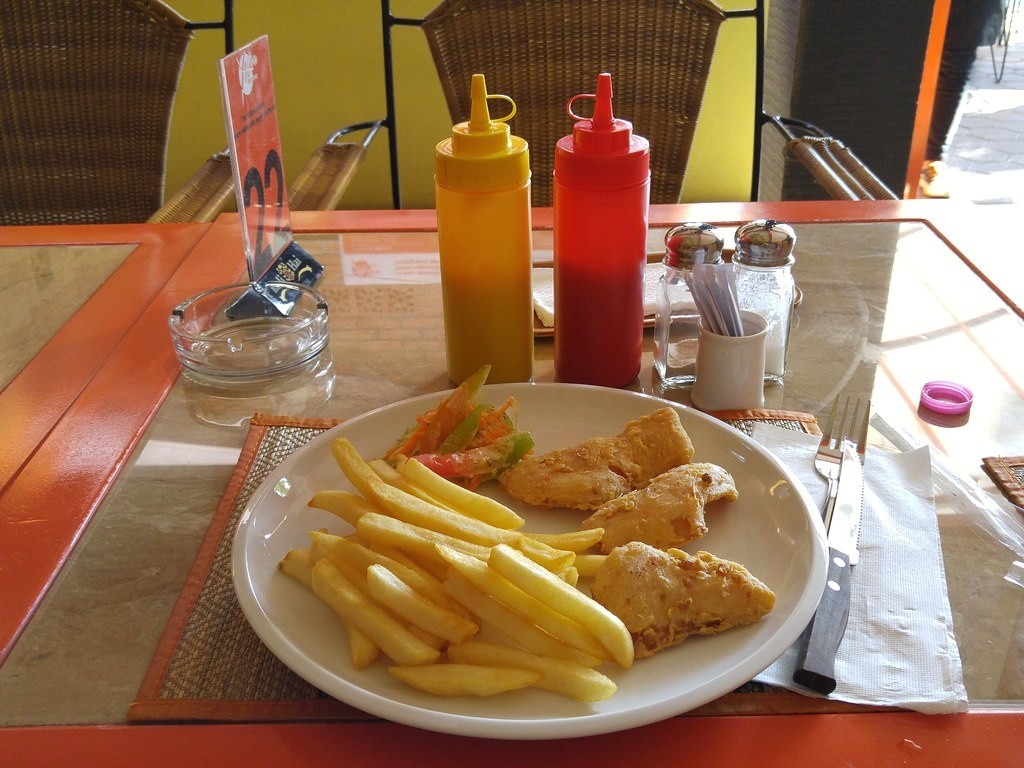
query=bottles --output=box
[653,219,795,384]
[435,74,535,386]
[553,73,651,388]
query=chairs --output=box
[0,0,237,224]
[286,0,901,211]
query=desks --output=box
[0,201,1024,767]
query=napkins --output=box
[745,422,969,716]
[530,261,699,329]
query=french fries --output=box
[274,438,634,704]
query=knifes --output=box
[793,443,864,695]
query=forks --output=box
[815,393,871,531]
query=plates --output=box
[231,383,829,740]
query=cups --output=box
[692,310,769,411]
